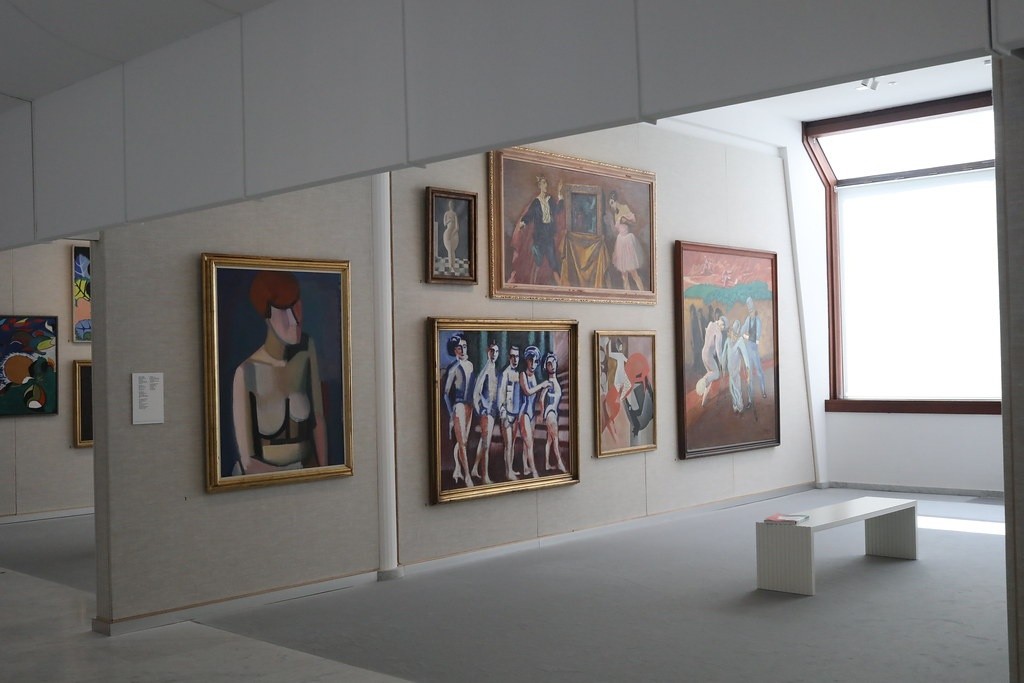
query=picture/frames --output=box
[593,330,658,458]
[427,315,581,506]
[424,186,479,285]
[0,315,59,418]
[674,239,781,462]
[199,251,356,495]
[487,146,658,305]
[73,359,94,449]
[71,243,91,344]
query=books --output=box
[764,513,810,525]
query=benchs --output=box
[756,496,918,595]
